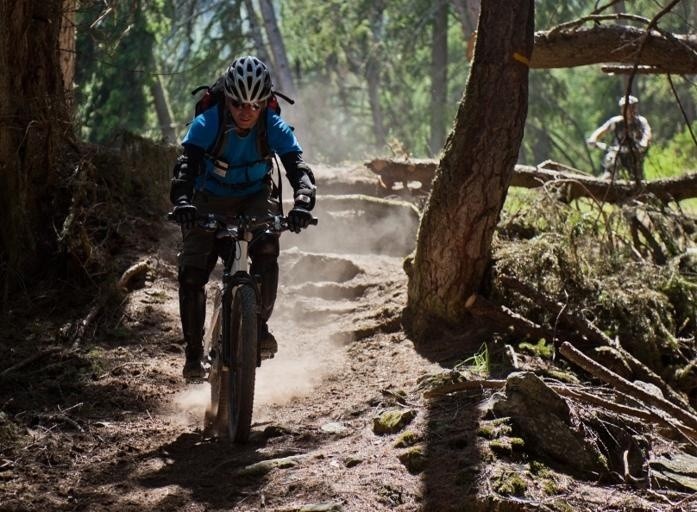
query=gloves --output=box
[287,205,313,233]
[171,201,199,229]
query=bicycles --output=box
[595,141,640,183]
[166,212,317,444]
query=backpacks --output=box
[195,76,280,158]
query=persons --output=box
[170,55,318,380]
[585,96,653,180]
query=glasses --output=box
[230,99,264,112]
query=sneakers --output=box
[259,324,277,353]
[183,343,204,377]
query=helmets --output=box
[617,95,639,108]
[224,55,272,101]
